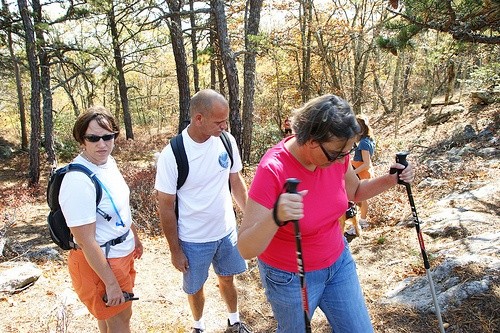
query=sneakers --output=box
[346,223,362,236]
[223,318,251,333]
[358,216,369,229]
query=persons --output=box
[238,94,416,333]
[333,185,364,238]
[154,89,258,333]
[352,113,376,229]
[57,105,143,333]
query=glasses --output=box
[82,133,116,142]
[314,136,358,162]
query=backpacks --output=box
[45,163,103,250]
